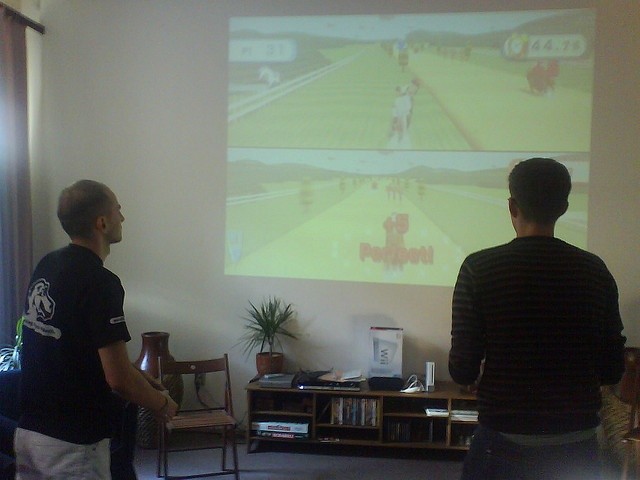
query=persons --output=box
[13,180,178,480]
[448,157,626,480]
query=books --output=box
[451,410,479,421]
[383,416,411,442]
[330,396,377,427]
[424,407,449,417]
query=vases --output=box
[134,331,185,452]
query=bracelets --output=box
[159,397,168,414]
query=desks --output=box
[242,377,488,455]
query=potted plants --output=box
[232,295,299,378]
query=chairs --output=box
[156,353,238,479]
